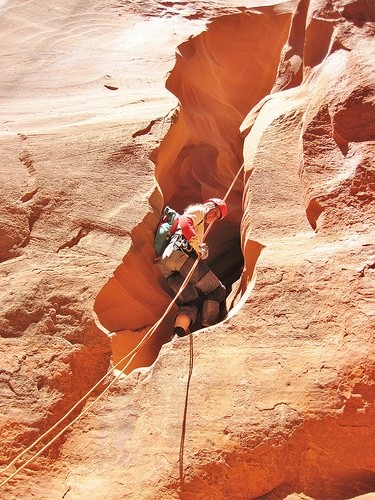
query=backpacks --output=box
[152,203,182,258]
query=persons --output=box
[156,197,230,340]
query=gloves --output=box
[190,234,210,260]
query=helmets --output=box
[207,197,228,221]
